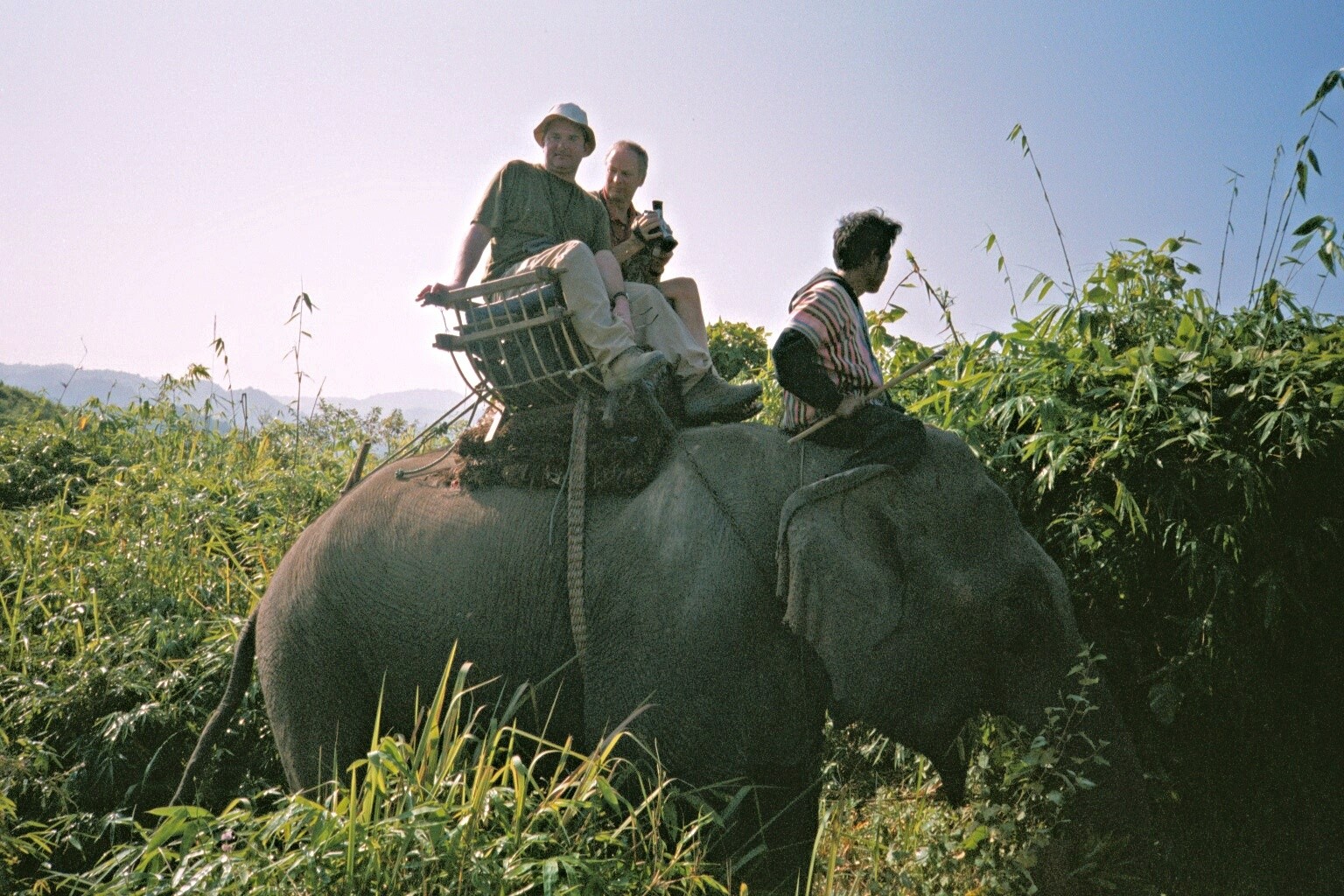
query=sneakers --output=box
[600,346,666,392]
[682,367,763,425]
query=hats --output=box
[533,103,596,157]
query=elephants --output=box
[155,424,1080,896]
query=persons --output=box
[580,138,762,423]
[773,206,931,472]
[415,103,763,428]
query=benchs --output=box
[415,265,677,414]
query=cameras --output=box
[648,200,679,253]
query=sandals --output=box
[711,402,764,423]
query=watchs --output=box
[611,290,630,306]
